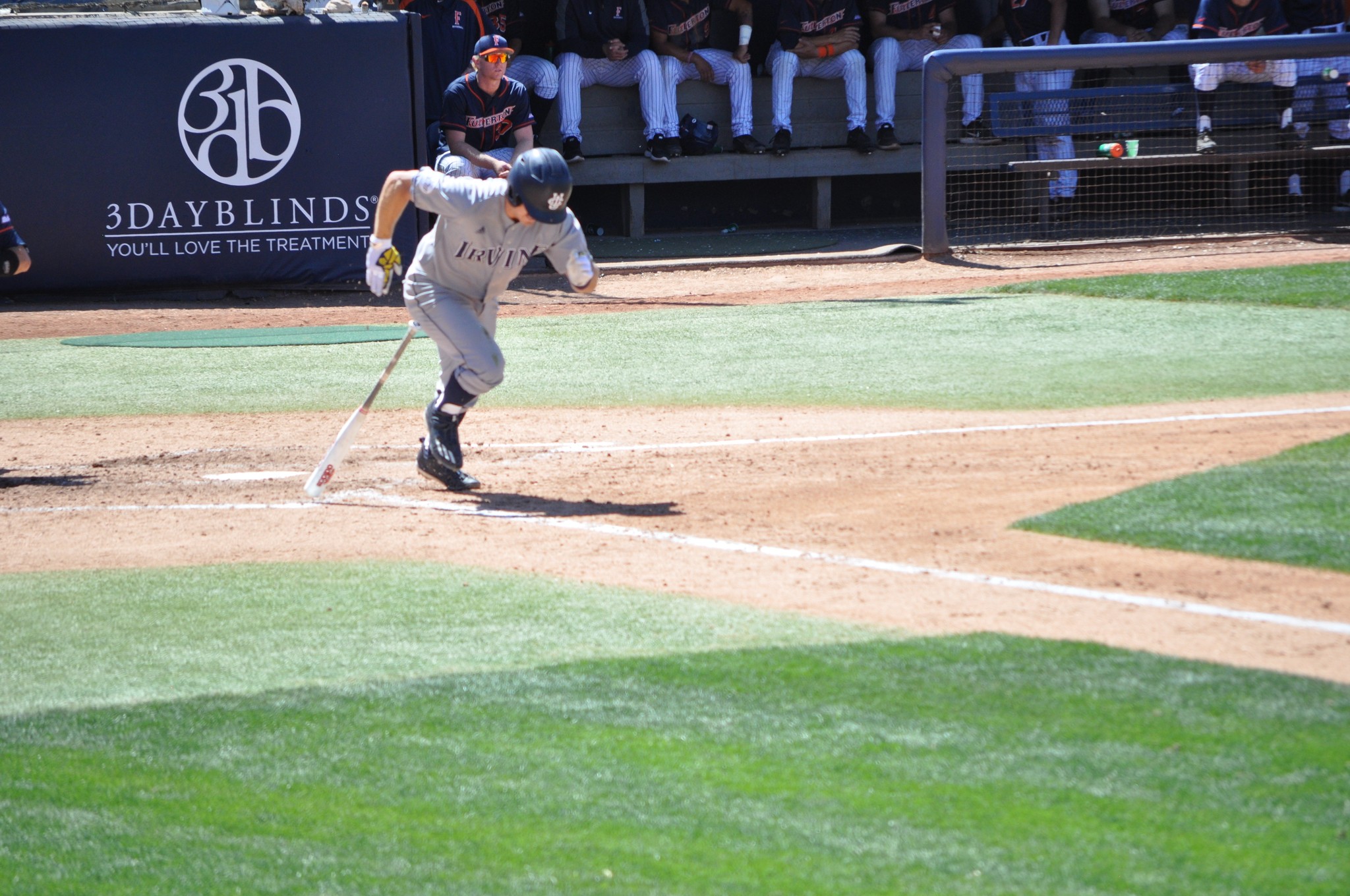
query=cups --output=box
[1125,140,1139,158]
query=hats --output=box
[474,34,514,56]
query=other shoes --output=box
[1286,193,1305,215]
[1331,191,1350,212]
[532,136,544,148]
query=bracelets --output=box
[739,24,752,45]
[687,52,693,63]
[817,44,835,58]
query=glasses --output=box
[480,53,508,64]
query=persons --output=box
[764,0,874,153]
[554,0,672,163]
[1188,0,1310,153]
[642,0,768,155]
[395,0,560,178]
[364,146,600,492]
[0,201,31,277]
[1080,0,1190,139]
[871,0,1078,221]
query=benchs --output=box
[531,65,1350,237]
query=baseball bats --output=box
[304,320,421,498]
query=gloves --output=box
[566,249,594,286]
[365,234,403,297]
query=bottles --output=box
[1096,144,1124,158]
[1322,68,1339,81]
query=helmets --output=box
[678,113,719,156]
[506,147,573,224]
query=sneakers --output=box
[876,123,901,149]
[1279,128,1312,149]
[732,134,766,154]
[1196,131,1216,154]
[425,390,462,472]
[1020,195,1073,220]
[563,136,585,163]
[769,126,791,156]
[416,437,480,491]
[644,133,671,162]
[846,125,875,153]
[959,115,1002,144]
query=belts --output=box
[1018,34,1046,46]
